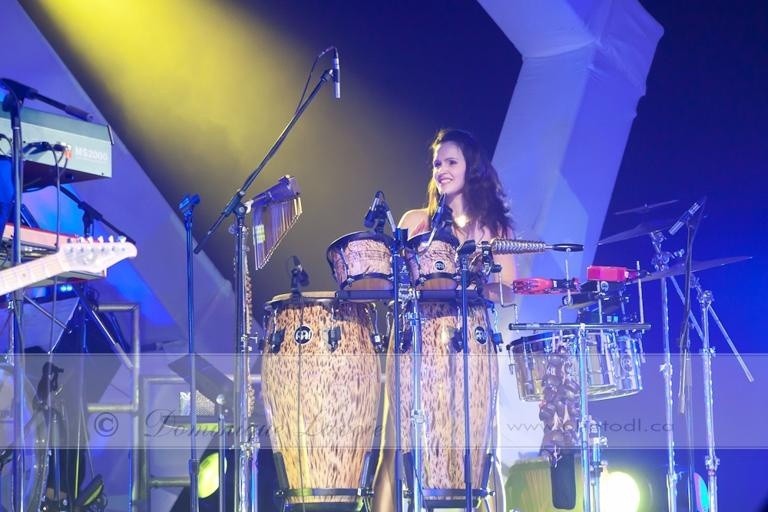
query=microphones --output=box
[431,192,445,229]
[291,255,310,287]
[332,49,341,100]
[460,253,482,289]
[364,192,380,228]
[668,198,705,236]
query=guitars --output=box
[0,236,138,298]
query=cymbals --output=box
[622,254,754,287]
[612,199,678,216]
[595,212,708,248]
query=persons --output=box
[370,127,517,512]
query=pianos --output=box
[0,101,114,188]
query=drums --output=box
[506,327,643,402]
[386,295,499,508]
[402,230,460,292]
[326,231,394,304]
[263,291,382,512]
